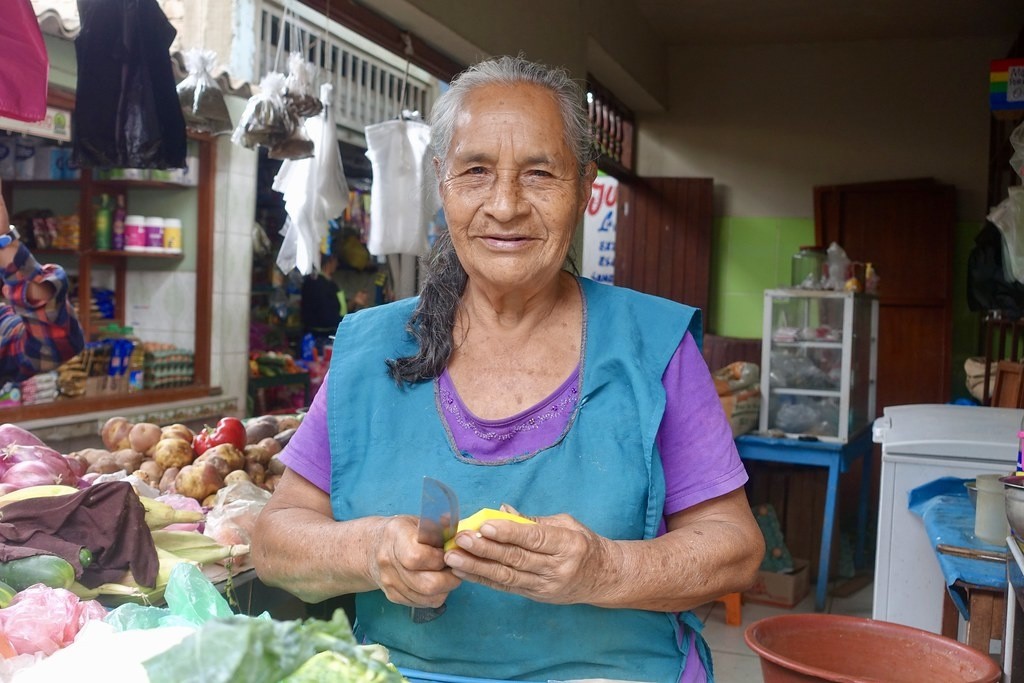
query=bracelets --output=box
[0,225,21,248]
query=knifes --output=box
[410,475,460,624]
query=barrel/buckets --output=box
[744,613,1002,683]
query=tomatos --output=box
[194,417,248,454]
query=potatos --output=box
[70,414,306,507]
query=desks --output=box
[940,580,1003,655]
[734,427,873,613]
[1001,536,1024,683]
[249,372,310,416]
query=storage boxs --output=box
[742,557,812,610]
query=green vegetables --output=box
[140,606,409,682]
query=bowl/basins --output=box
[998,475,1024,542]
[974,473,1008,547]
[964,482,977,511]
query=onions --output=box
[0,421,92,496]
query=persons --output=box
[0,193,86,382]
[252,49,766,683]
[300,251,369,354]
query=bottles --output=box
[789,246,828,289]
[123,214,145,252]
[164,218,182,255]
[113,194,127,251]
[145,217,165,254]
[94,193,112,251]
[96,322,145,392]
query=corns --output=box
[0,484,251,605]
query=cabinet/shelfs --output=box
[0,166,190,346]
[758,288,879,444]
